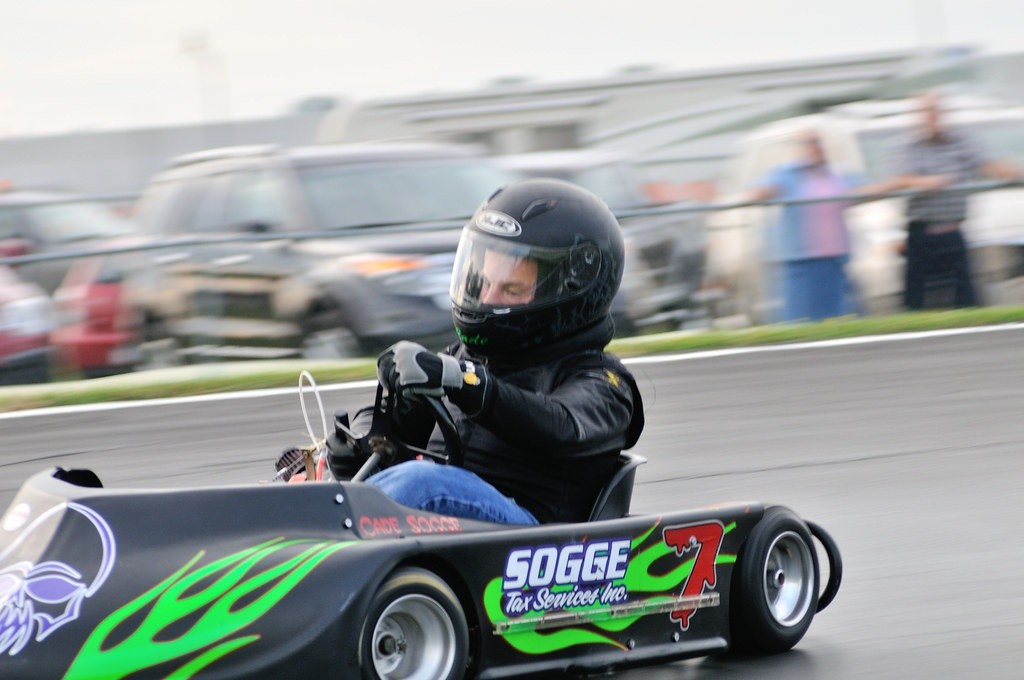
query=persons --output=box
[750,127,878,324]
[874,90,1023,307]
[324,177,642,522]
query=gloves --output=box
[325,432,360,482]
[377,339,494,420]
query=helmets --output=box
[450,177,626,372]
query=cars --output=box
[45,73,1024,385]
[1,183,154,387]
